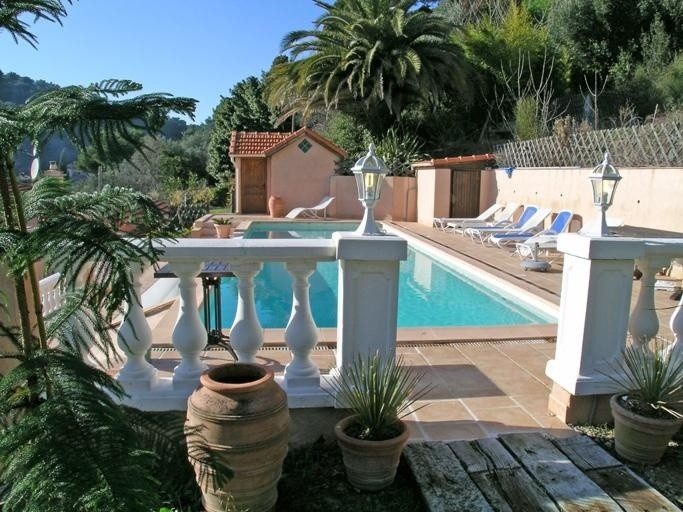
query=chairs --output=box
[433,203,573,262]
[285,195,336,219]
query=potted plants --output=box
[318,346,441,490]
[593,334,683,466]
[211,217,233,238]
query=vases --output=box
[269,195,283,218]
[183,362,290,512]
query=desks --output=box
[154,257,238,362]
